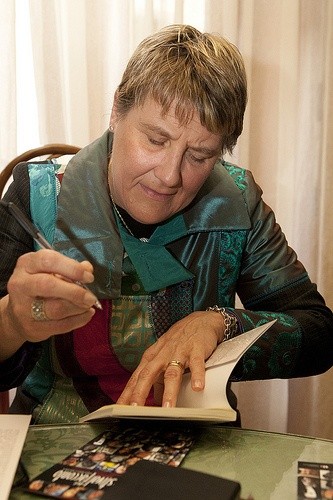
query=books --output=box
[77,317,278,423]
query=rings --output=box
[30,297,49,321]
[167,360,186,374]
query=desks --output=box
[7,422,333,500]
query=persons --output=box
[0,21,333,424]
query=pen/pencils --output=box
[9,203,103,311]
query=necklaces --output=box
[110,193,149,242]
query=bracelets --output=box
[205,304,232,342]
